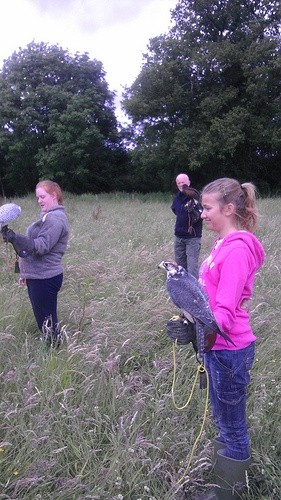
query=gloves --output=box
[167,316,218,353]
[184,204,200,224]
[0,224,35,258]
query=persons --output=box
[0,181,69,350]
[158,179,265,500]
[170,174,203,278]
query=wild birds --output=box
[0,204,22,231]
[183,184,201,202]
[157,258,237,347]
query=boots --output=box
[213,450,250,500]
[204,438,227,480]
